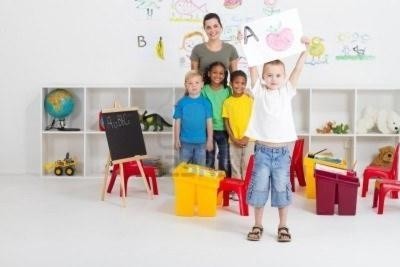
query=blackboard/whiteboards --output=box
[101,106,148,164]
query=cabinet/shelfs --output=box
[39,84,400,177]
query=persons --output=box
[189,13,238,89]
[236,31,309,242]
[198,61,232,198]
[172,69,214,165]
[221,70,254,201]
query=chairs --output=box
[372,177,400,214]
[107,158,160,197]
[361,142,400,197]
[219,153,256,217]
[289,138,307,192]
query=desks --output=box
[304,152,349,199]
[172,161,230,218]
[312,162,359,216]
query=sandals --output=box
[277,227,292,243]
[247,225,264,241]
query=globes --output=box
[44,89,81,131]
